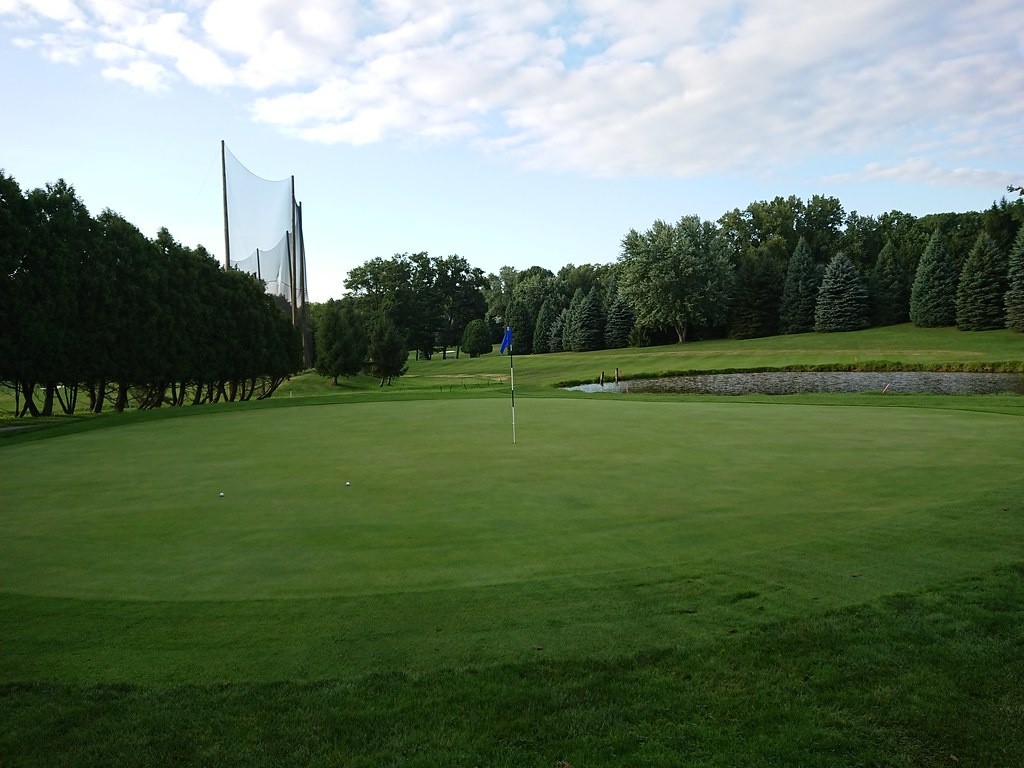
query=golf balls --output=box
[344,481,351,486]
[219,493,224,497]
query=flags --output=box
[500,327,512,355]
